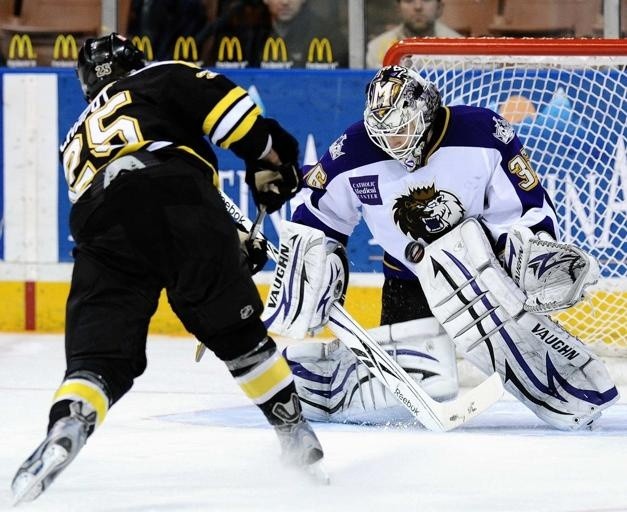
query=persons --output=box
[365,1,472,71]
[120,1,350,70]
[9,33,329,503]
[280,61,624,430]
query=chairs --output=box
[0,0,625,69]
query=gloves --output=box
[245,118,302,214]
[232,222,267,274]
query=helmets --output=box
[363,65,441,138]
[77,33,146,98]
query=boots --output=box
[12,416,86,507]
[277,427,323,465]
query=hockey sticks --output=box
[221,186,505,436]
[191,185,282,364]
